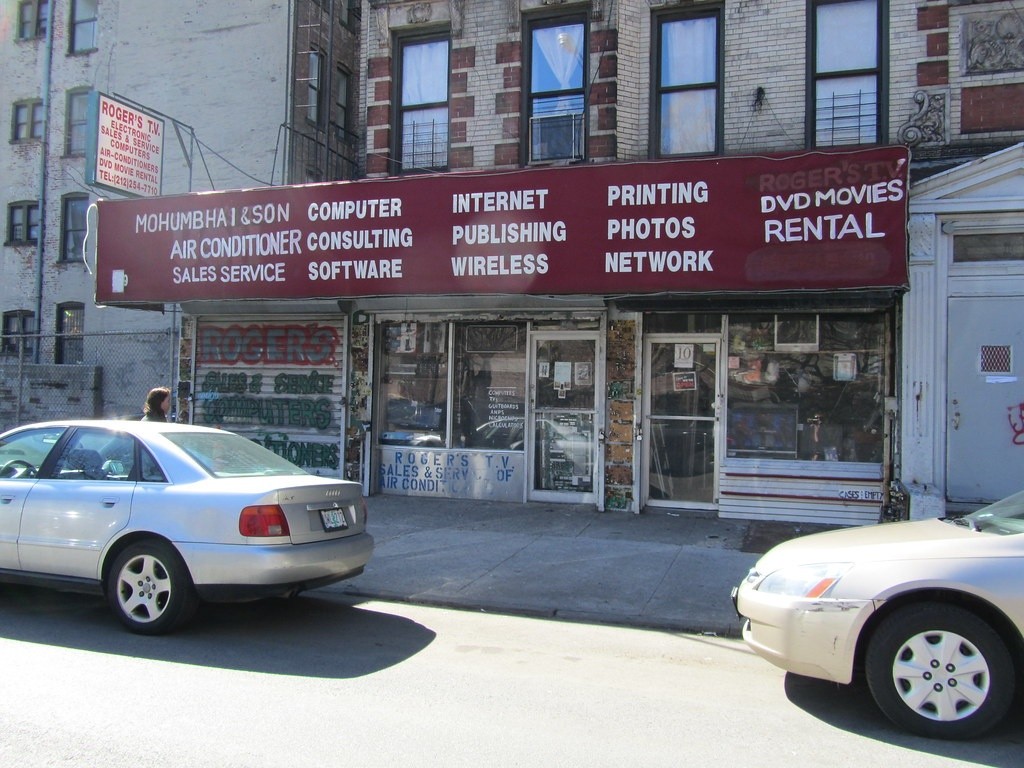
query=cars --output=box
[0,420,378,639]
[730,490,1024,742]
[407,416,595,483]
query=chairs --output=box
[69,449,103,480]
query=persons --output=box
[140,387,171,422]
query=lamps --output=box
[336,298,357,315]
[111,268,127,293]
